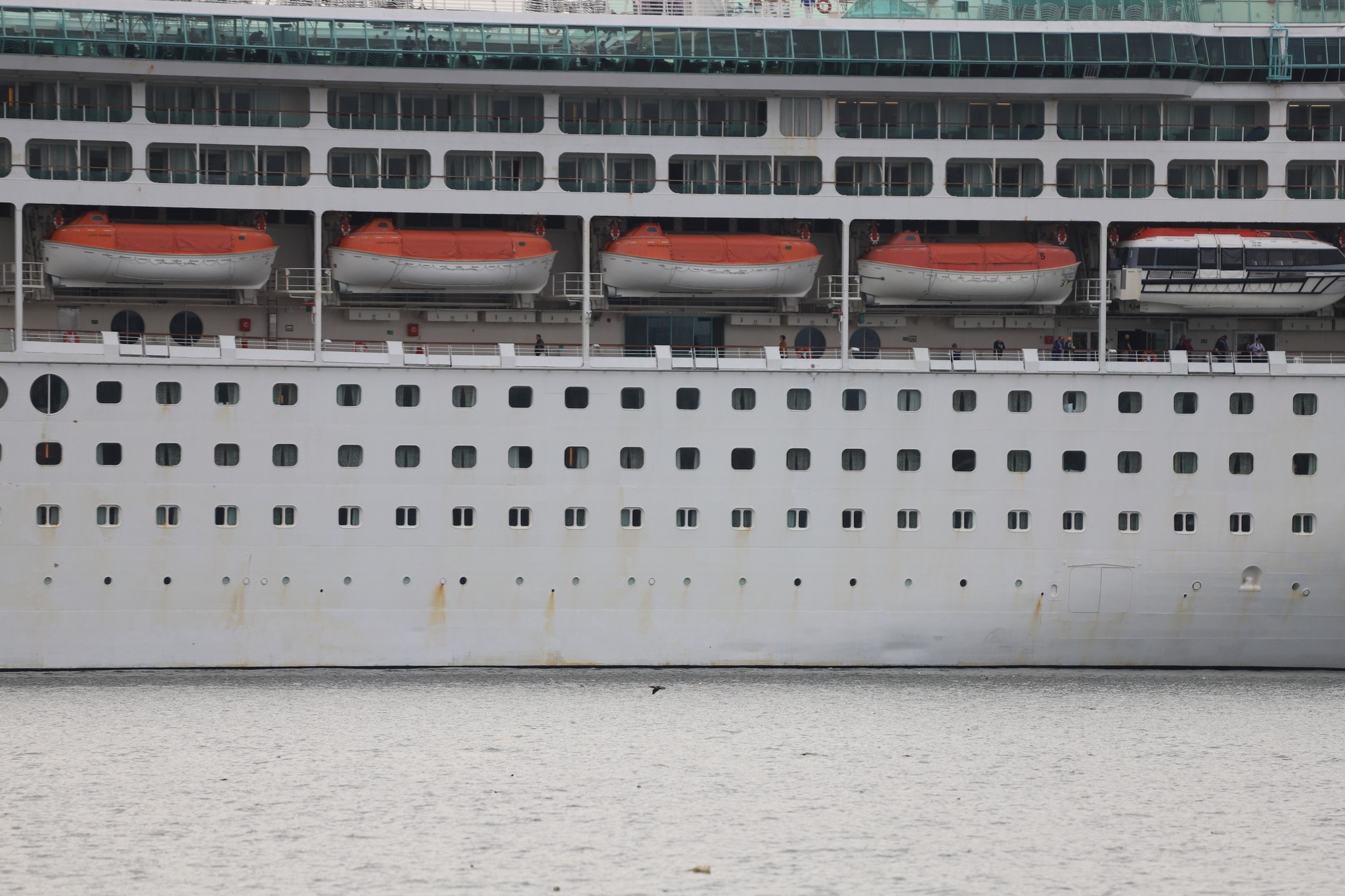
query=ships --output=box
[0,0,1345,673]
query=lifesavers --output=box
[353,342,368,352]
[1141,351,1157,362]
[63,331,80,343]
[798,347,813,359]
[816,1,832,13]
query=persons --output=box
[1,5,1344,82]
[1043,331,1270,366]
[534,333,546,356]
[950,342,962,361]
[994,337,1006,360]
[779,335,788,359]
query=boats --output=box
[598,221,820,302]
[326,217,559,300]
[1107,226,1345,317]
[856,229,1080,309]
[36,208,279,290]
